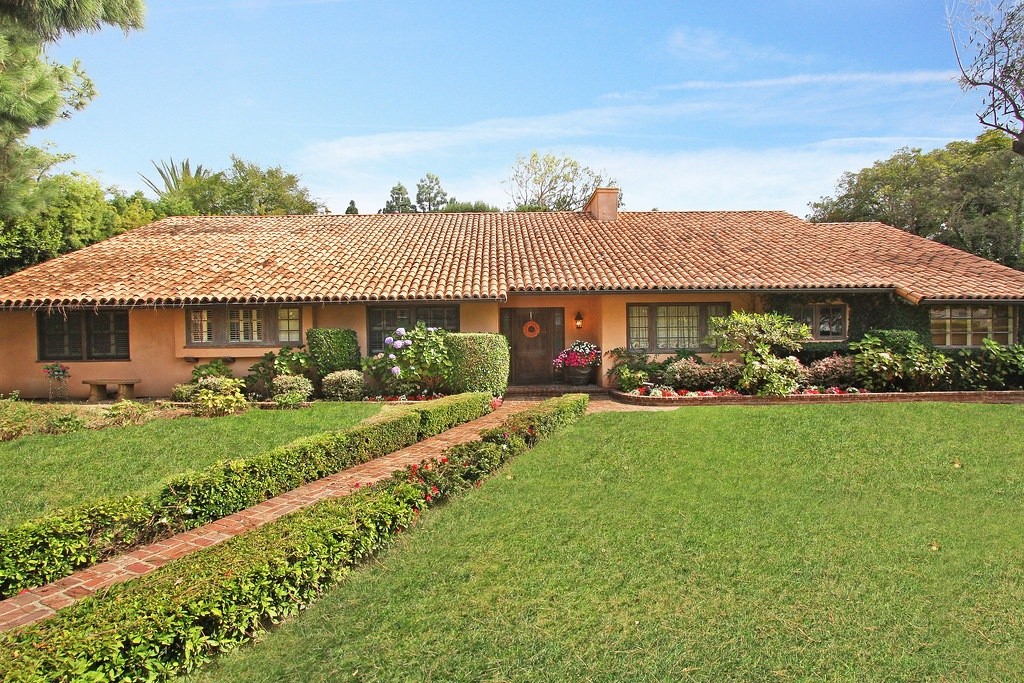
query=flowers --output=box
[553,339,601,373]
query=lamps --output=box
[574,310,584,329]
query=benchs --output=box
[82,379,142,403]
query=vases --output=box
[564,367,594,386]
